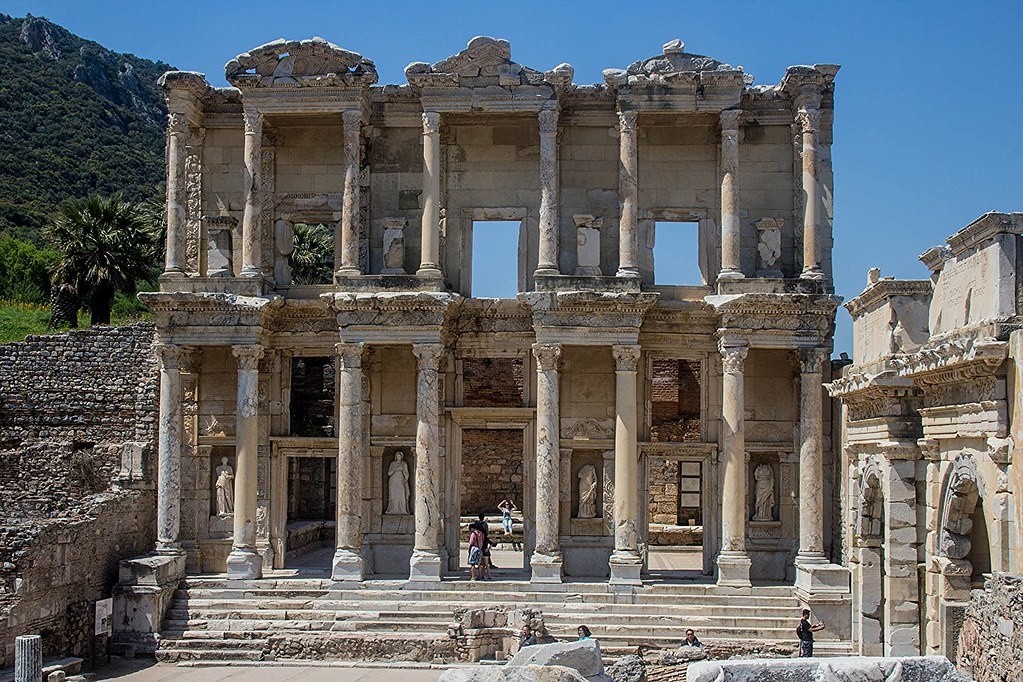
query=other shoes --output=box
[468,577,481,581]
[483,576,491,580]
[490,565,498,569]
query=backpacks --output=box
[796,621,804,640]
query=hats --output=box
[479,513,486,518]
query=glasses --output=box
[687,633,693,636]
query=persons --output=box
[682,629,703,647]
[215,456,234,521]
[387,452,409,513]
[578,464,600,519]
[469,513,498,581]
[518,625,535,651]
[754,463,774,519]
[577,625,591,642]
[801,609,826,657]
[497,499,518,535]
